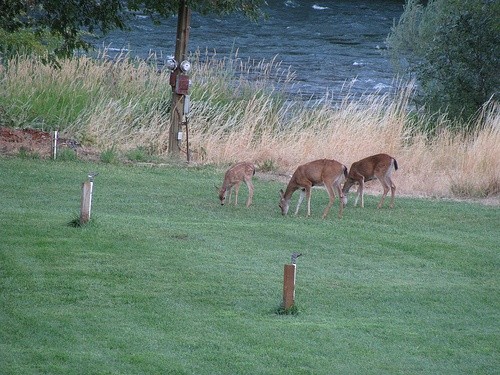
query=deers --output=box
[278,158,348,221]
[342,152,398,209]
[214,160,255,207]
